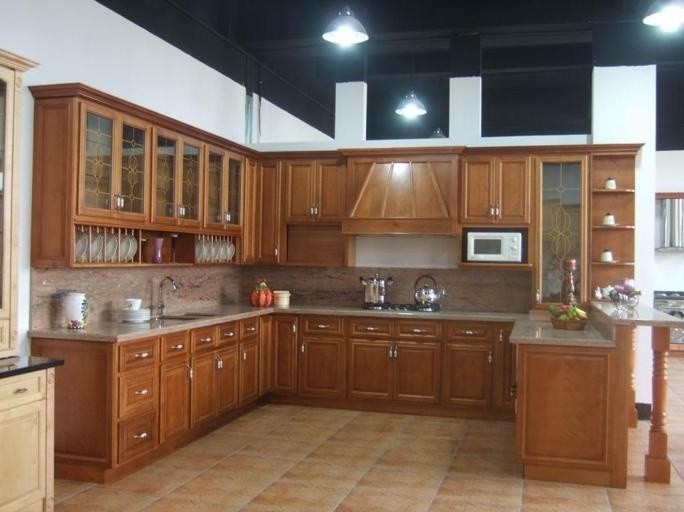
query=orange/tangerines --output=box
[559,314,568,321]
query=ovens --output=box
[654,297,684,344]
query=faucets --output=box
[157,277,177,315]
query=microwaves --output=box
[464,231,523,263]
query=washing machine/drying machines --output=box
[655,290,684,344]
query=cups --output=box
[150,237,164,264]
[62,291,88,329]
[126,298,141,309]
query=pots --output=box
[360,272,393,305]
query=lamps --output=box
[642,1,683,28]
[393,88,428,118]
[320,1,369,47]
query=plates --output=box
[74,228,138,263]
[196,238,235,261]
[119,308,150,324]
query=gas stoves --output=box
[359,301,440,312]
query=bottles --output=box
[603,213,615,226]
[600,248,613,262]
[592,284,614,299]
[603,177,616,189]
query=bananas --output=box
[567,306,588,320]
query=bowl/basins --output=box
[551,317,587,331]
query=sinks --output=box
[158,316,196,321]
[175,313,218,317]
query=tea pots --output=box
[413,274,445,306]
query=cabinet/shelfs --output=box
[297,316,346,400]
[257,160,280,264]
[494,322,513,406]
[0,370,47,511]
[190,321,239,428]
[30,96,150,223]
[281,159,346,225]
[442,320,494,406]
[0,64,22,359]
[31,223,139,268]
[197,232,243,266]
[588,152,636,305]
[347,320,442,401]
[509,344,613,470]
[150,123,202,227]
[239,318,260,406]
[461,155,535,225]
[160,328,190,446]
[535,154,587,310]
[30,335,160,470]
[140,230,198,267]
[273,314,297,395]
[201,140,244,231]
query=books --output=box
[655,198,684,248]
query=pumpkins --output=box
[250,282,272,307]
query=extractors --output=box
[654,200,683,250]
